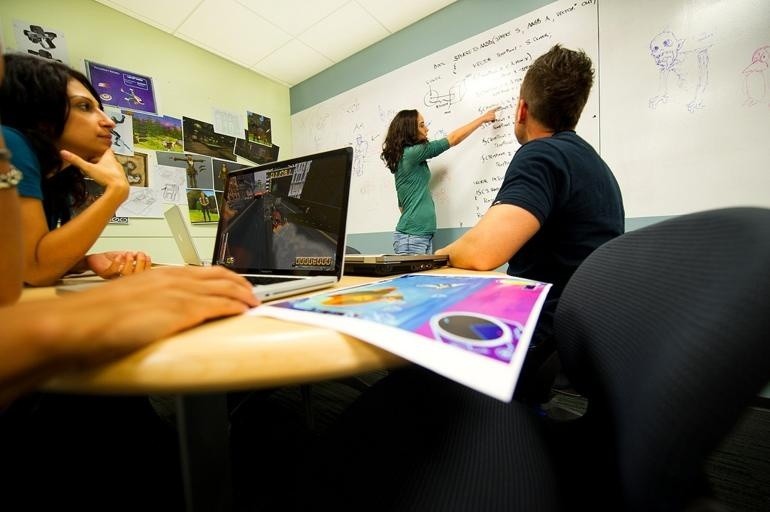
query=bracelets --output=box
[0,148,24,190]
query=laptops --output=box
[210,146,353,304]
[164,204,212,266]
[344,254,448,277]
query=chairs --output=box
[318,209,767,512]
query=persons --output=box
[431,46,626,302]
[217,162,229,185]
[198,192,212,223]
[380,105,502,256]
[172,156,203,188]
[0,131,262,512]
[0,52,152,288]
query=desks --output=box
[2,260,515,508]
